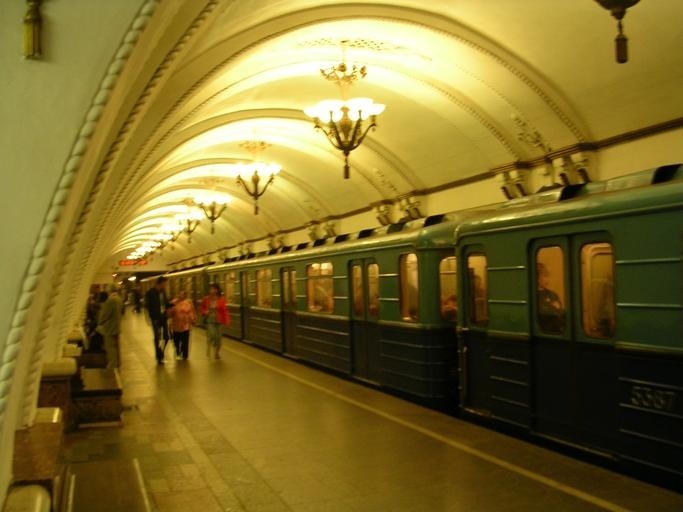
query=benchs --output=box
[3,330,153,512]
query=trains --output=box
[138,163,681,492]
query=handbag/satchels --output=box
[195,308,208,329]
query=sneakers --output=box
[157,354,221,362]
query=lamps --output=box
[302,42,387,179]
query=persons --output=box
[443,294,457,321]
[83,275,232,373]
[536,260,565,336]
[473,276,486,323]
